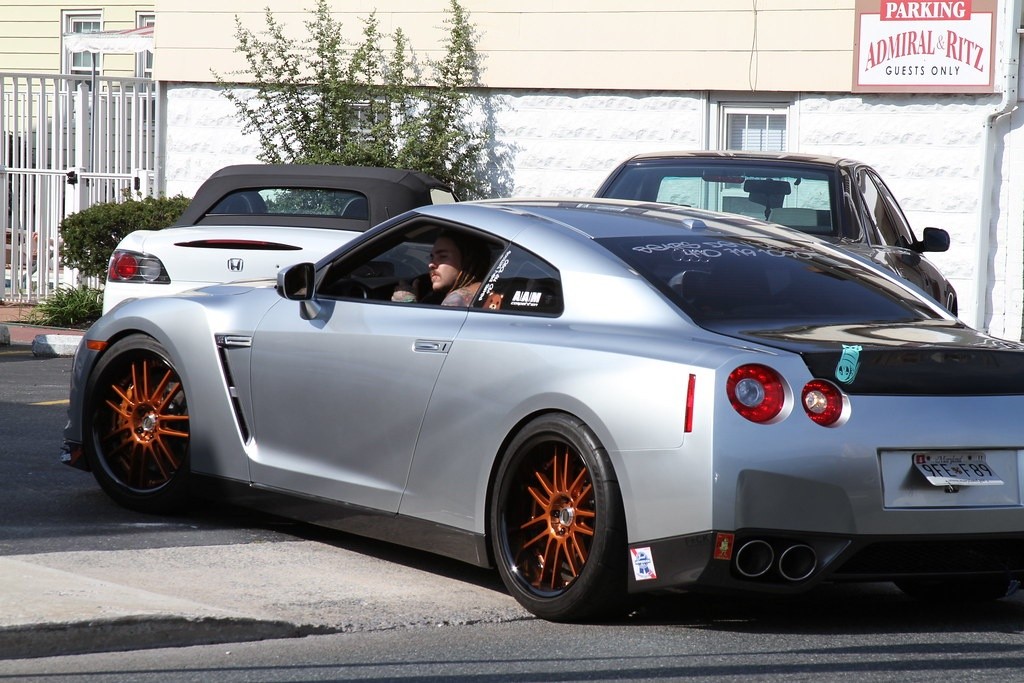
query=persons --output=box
[391,232,493,316]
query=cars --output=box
[590,150,961,320]
[100,162,462,319]
[66,196,1023,625]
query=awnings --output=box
[64,25,155,55]
